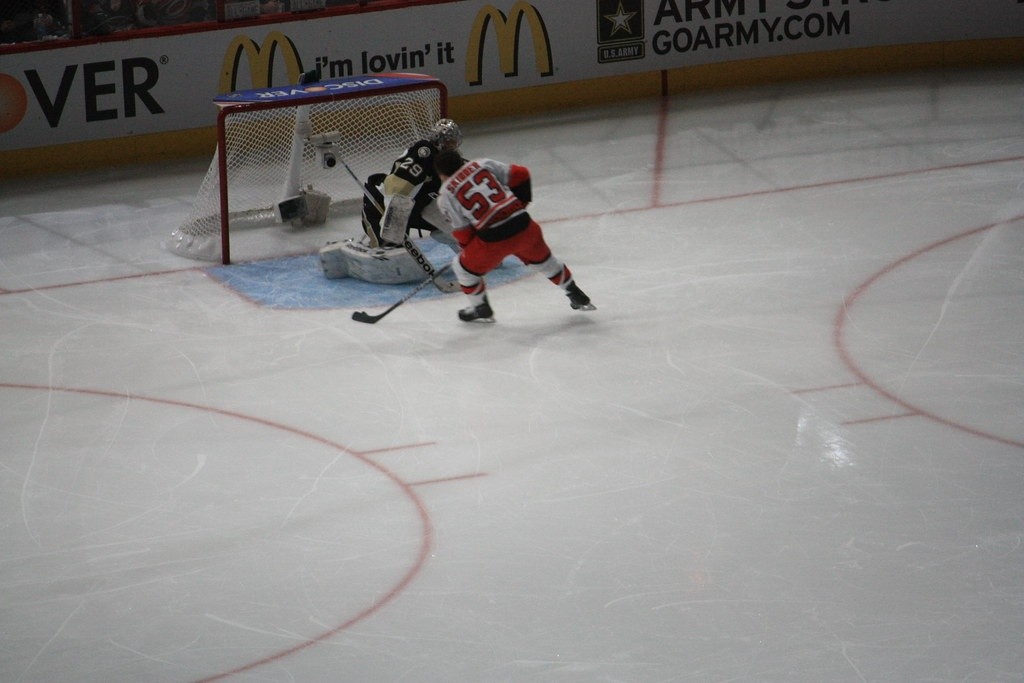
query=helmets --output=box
[430,118,464,155]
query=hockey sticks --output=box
[350,260,454,326]
[327,144,464,296]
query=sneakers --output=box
[458,296,493,321]
[566,280,597,310]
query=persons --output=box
[362,118,597,321]
